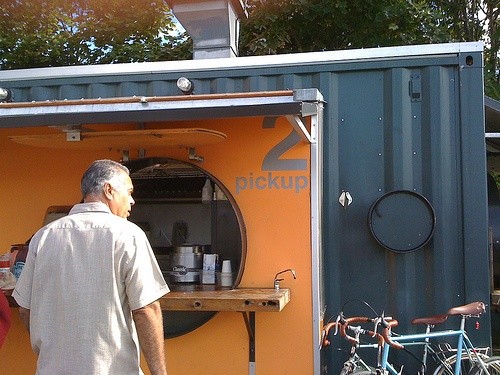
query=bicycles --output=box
[333,302,500,375]
[316,317,490,375]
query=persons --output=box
[11,159,170,375]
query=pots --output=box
[171,244,210,271]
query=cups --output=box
[0,254,10,272]
[221,260,233,274]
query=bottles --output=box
[202,179,212,202]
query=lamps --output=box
[0,88,14,102]
[176,76,195,96]
